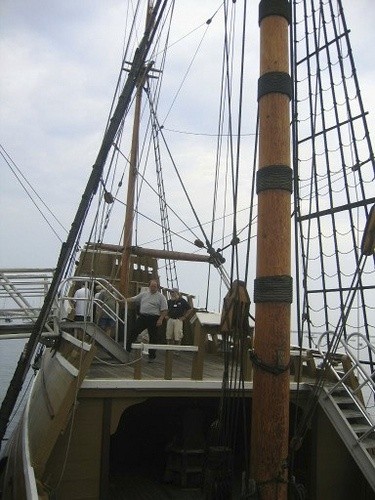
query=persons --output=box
[166,288,194,358]
[118,279,169,363]
[97,285,116,338]
[72,280,93,322]
[93,285,105,326]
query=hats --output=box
[170,288,178,294]
[96,285,102,290]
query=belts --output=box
[141,314,159,317]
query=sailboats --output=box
[0,0,375,500]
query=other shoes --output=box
[147,359,156,363]
[174,352,179,356]
